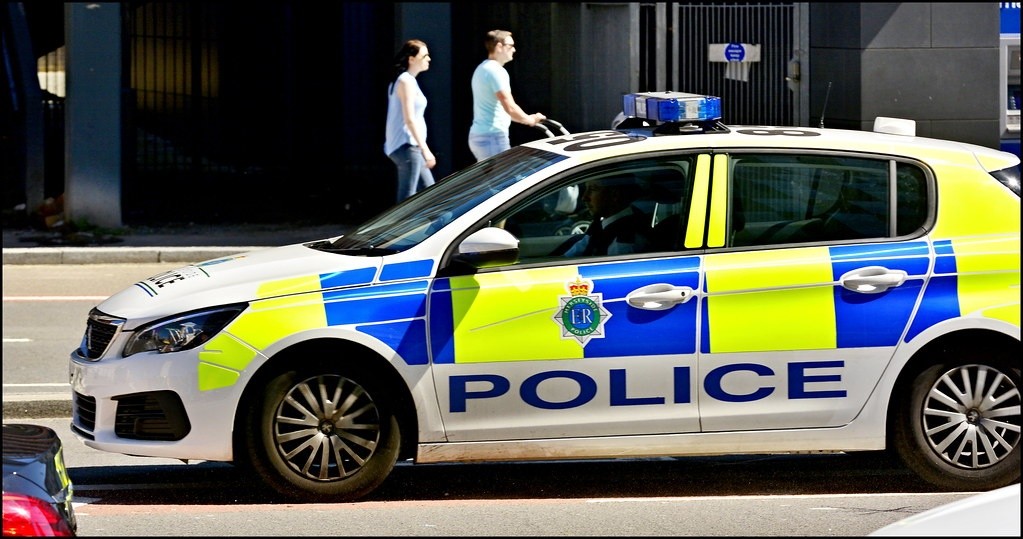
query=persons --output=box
[467,29,547,228]
[559,172,647,259]
[383,39,437,204]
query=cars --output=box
[2,424,77,537]
[71,89,1021,501]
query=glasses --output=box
[503,44,514,49]
[420,54,429,59]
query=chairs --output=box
[638,214,686,253]
